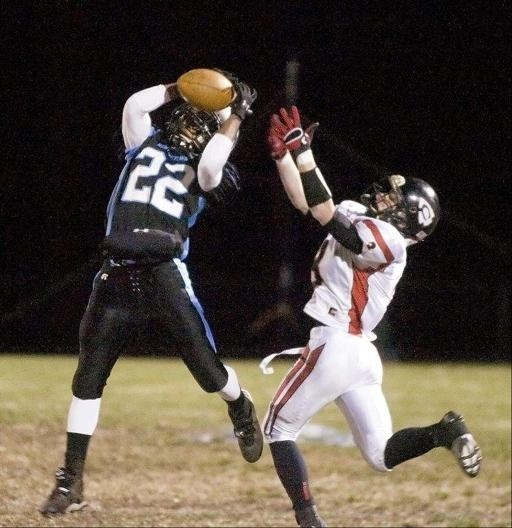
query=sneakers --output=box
[229,389,263,462]
[39,468,88,515]
[441,411,482,477]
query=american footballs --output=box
[176,68,237,111]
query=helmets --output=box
[360,174,441,241]
[164,103,240,159]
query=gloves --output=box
[213,68,257,120]
[268,106,320,162]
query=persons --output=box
[38,66,264,517]
[263,105,485,527]
